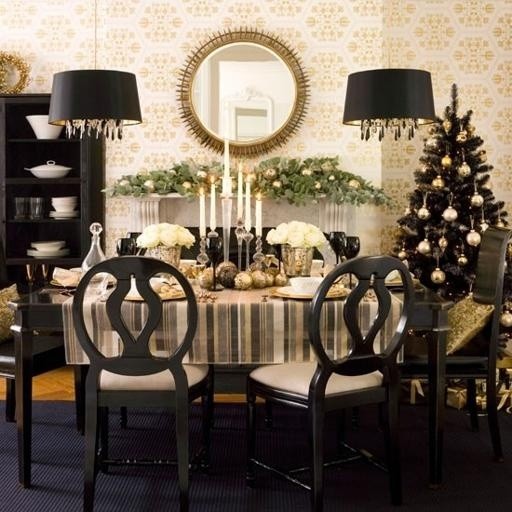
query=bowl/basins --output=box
[290,277,325,294]
[25,115,64,140]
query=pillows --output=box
[442,293,494,356]
[1,286,21,344]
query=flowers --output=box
[266,220,327,249]
[136,223,198,250]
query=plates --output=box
[368,278,417,288]
[270,286,351,300]
[50,278,114,288]
[47,196,79,220]
[124,290,186,302]
[26,239,71,259]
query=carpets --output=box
[1,399,511,511]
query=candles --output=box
[255,191,263,236]
[245,175,252,233]
[223,137,231,193]
[235,159,244,219]
[209,175,217,231]
[197,186,208,238]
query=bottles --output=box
[80,222,110,300]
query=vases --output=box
[280,243,312,279]
[148,245,182,280]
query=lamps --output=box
[46,1,144,140]
[341,1,438,142]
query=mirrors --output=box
[177,27,313,161]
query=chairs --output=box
[73,254,214,511]
[384,225,512,487]
[244,255,416,511]
[0,235,81,488]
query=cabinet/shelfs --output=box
[1,93,107,292]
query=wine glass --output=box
[204,236,225,291]
[329,231,347,266]
[343,235,359,289]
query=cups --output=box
[179,258,205,283]
[116,231,148,257]
[311,260,323,276]
[14,196,44,220]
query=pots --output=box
[25,160,73,179]
